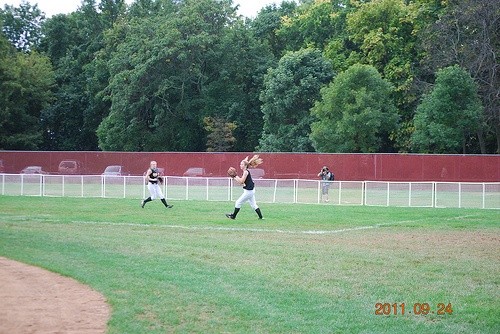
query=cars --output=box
[101,165,131,184]
[18,167,50,183]
[143,168,166,184]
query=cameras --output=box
[322,169,326,172]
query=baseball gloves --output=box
[227,167,237,178]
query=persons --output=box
[141,161,174,209]
[226,154,264,220]
[318,166,332,203]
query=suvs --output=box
[247,168,271,185]
[58,159,86,182]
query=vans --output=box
[183,168,213,185]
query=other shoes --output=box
[140,202,145,208]
[166,204,173,208]
[226,212,235,218]
[258,215,264,218]
